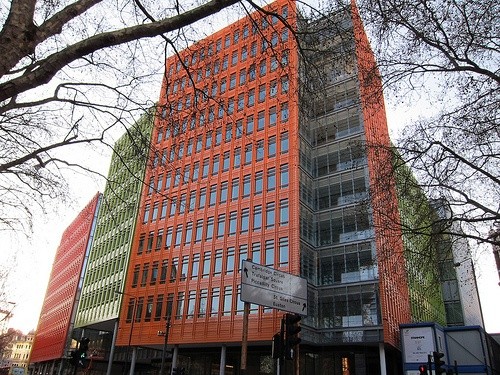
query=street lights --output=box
[156,314,172,372]
[114,290,137,375]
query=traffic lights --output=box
[438,353,447,374]
[419,365,428,375]
[77,352,87,368]
[285,313,302,362]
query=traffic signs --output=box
[241,259,309,316]
